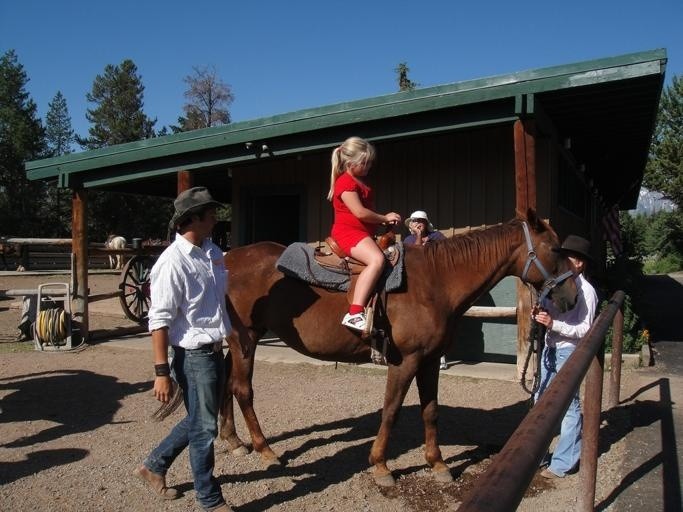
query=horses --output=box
[150,207,578,487]
[0,237,25,271]
[104,234,128,270]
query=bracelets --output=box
[153,362,169,376]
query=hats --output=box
[561,234,595,261]
[404,209,434,230]
[168,185,228,226]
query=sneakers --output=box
[539,463,565,478]
[340,310,378,336]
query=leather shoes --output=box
[134,464,180,500]
[204,503,234,512]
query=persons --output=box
[401,209,448,371]
[131,185,254,511]
[531,234,599,481]
[324,135,402,334]
